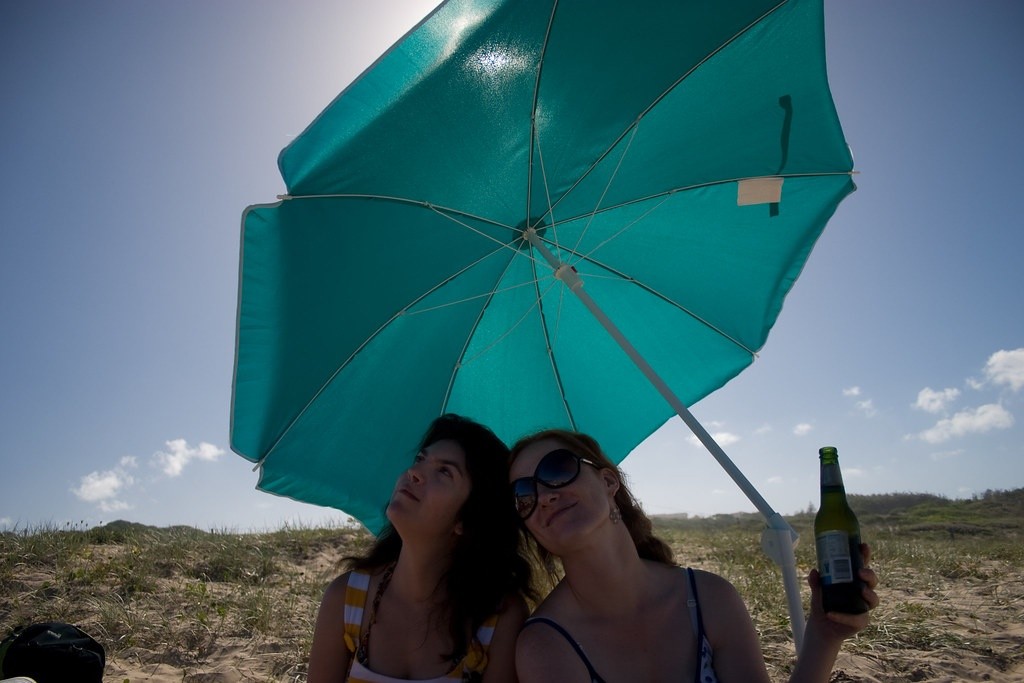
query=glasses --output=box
[507,448,601,523]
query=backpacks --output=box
[0,623,105,683]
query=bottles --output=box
[814,447,869,615]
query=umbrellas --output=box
[228,0,858,659]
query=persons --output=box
[307,413,561,683]
[511,430,879,683]
